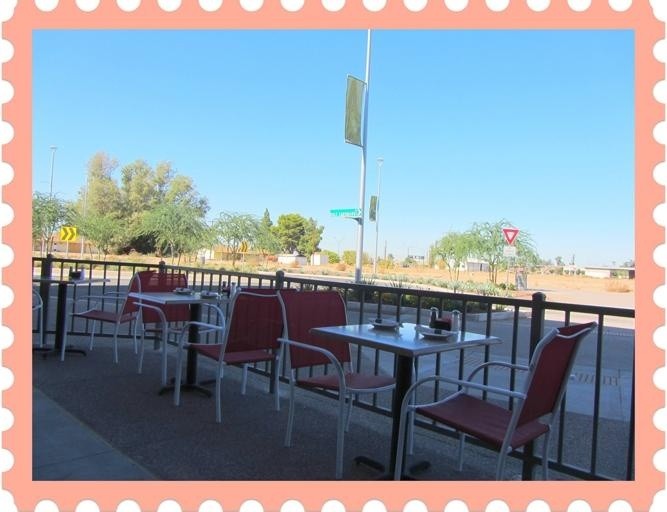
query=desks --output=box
[373,157,386,275]
[310,322,502,477]
[48,145,59,202]
[32,274,111,359]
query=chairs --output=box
[277,289,398,481]
[58,271,228,398]
[394,321,600,479]
[173,287,297,422]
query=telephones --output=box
[328,208,363,219]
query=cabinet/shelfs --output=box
[428,307,440,324]
[221,281,237,298]
[451,309,460,334]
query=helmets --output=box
[415,324,456,338]
[374,322,400,328]
[172,287,194,293]
[199,291,221,298]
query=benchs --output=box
[344,72,367,148]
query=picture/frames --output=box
[32,274,111,359]
